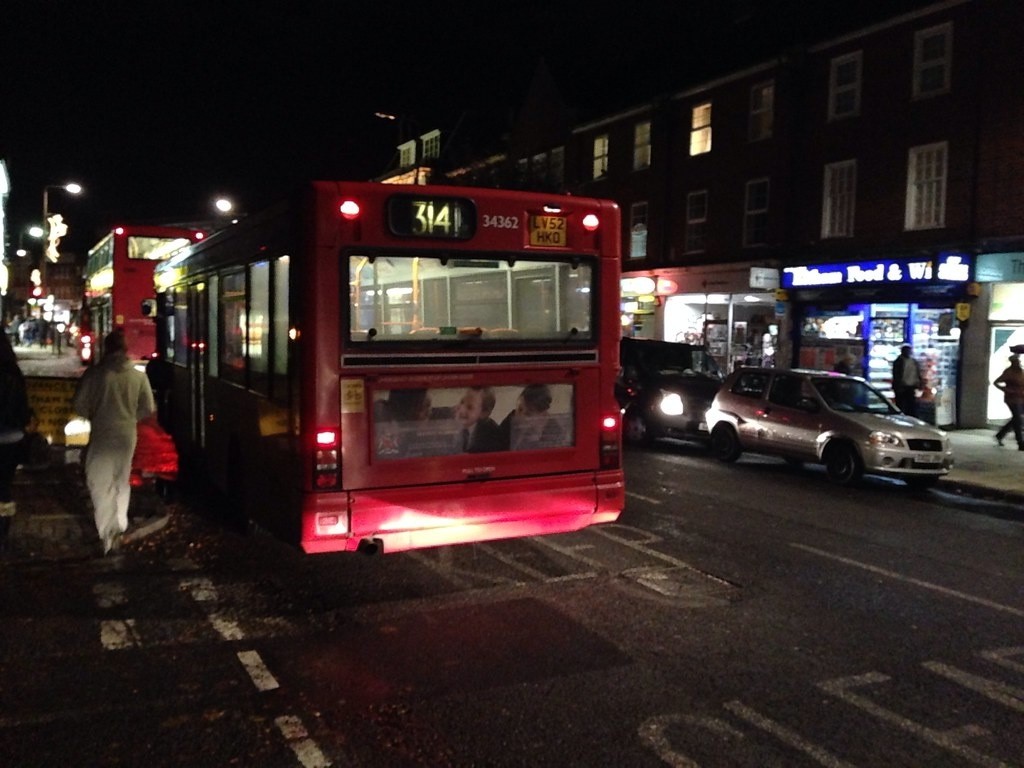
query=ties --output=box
[462,430,471,456]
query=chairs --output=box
[351,327,519,342]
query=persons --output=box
[381,384,565,455]
[0,325,31,539]
[72,329,158,558]
[701,345,1024,451]
[7,313,71,354]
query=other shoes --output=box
[1018,444,1024,451]
[103,530,125,557]
[996,436,1004,446]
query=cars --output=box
[708,365,956,486]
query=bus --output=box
[85,224,205,367]
[618,335,723,449]
[147,177,626,558]
